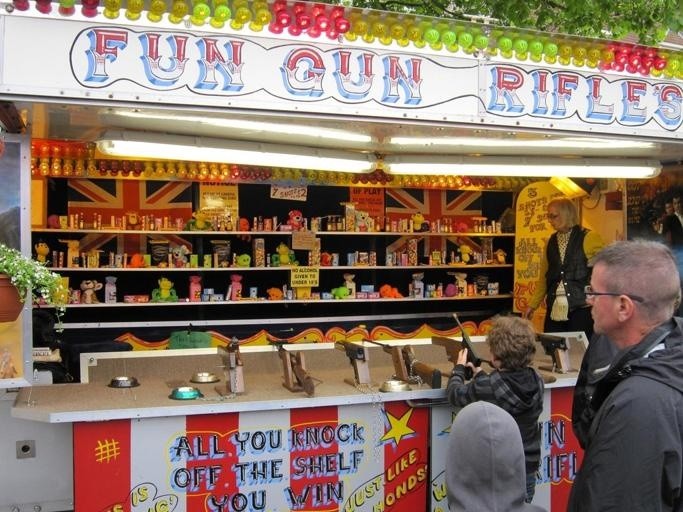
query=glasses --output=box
[584,284,643,303]
[548,214,559,218]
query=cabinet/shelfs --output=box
[29,228,514,310]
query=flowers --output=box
[0,244,72,334]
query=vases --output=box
[0,273,28,322]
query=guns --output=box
[266,335,314,395]
[334,341,375,384]
[452,313,482,381]
[217,337,242,368]
[362,338,441,390]
[535,332,567,367]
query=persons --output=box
[522,198,606,343]
[568,240,683,512]
[445,317,548,512]
[651,192,683,251]
